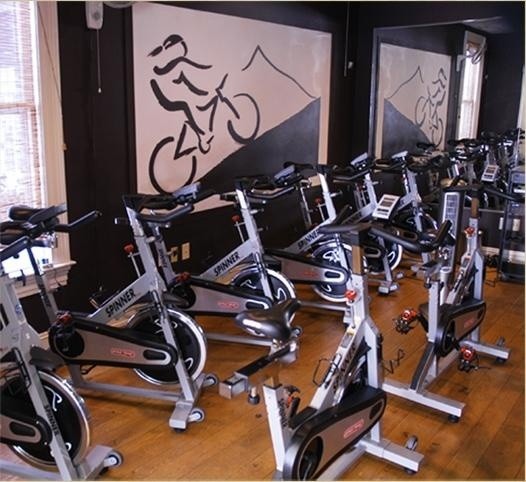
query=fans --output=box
[456,42,487,72]
[85,1,138,94]
